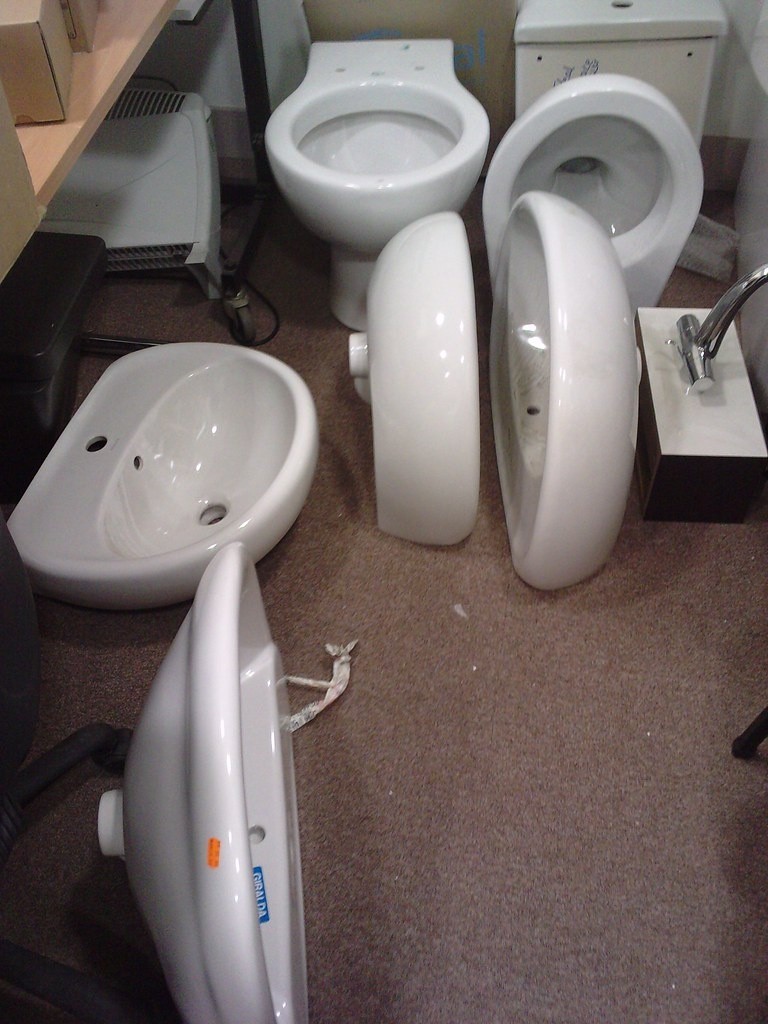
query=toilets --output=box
[510,0,729,179]
[481,73,704,306]
[264,37,492,331]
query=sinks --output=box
[346,210,481,549]
[489,189,641,591]
[120,538,312,1024]
[8,343,318,614]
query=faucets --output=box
[677,261,768,390]
